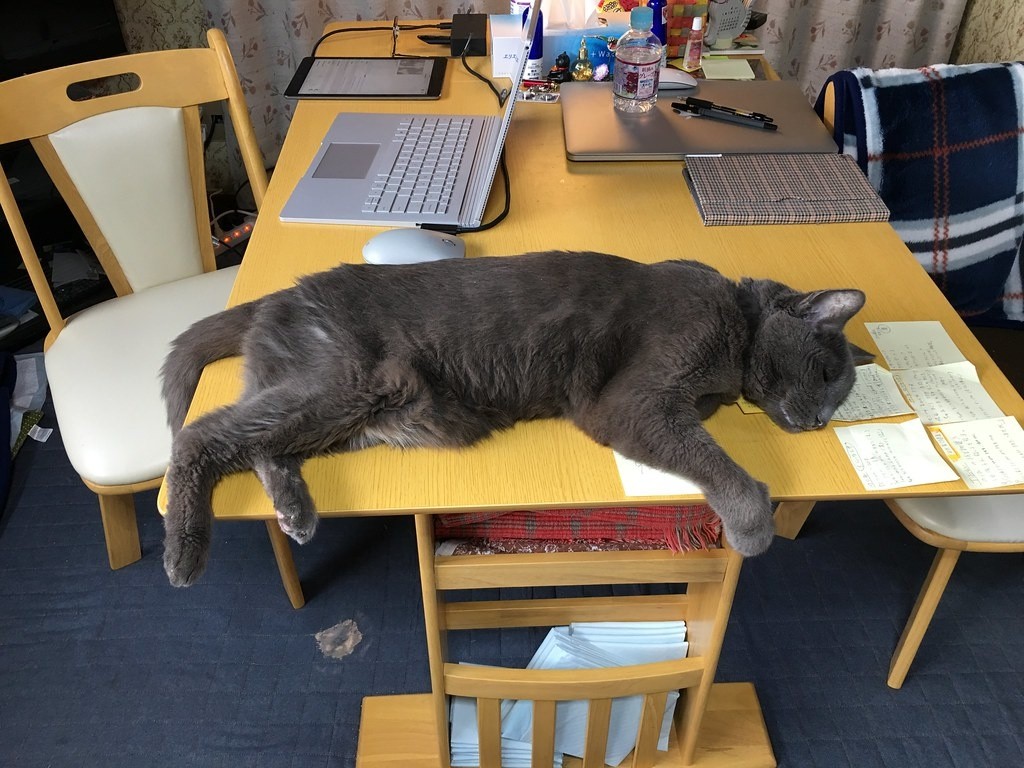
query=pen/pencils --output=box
[671,96,778,130]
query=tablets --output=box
[285,57,448,100]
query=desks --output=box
[158,19,1024,611]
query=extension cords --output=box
[212,219,257,256]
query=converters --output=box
[451,14,488,56]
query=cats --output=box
[154,247,876,591]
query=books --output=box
[448,618,689,767]
[50,247,106,289]
[682,153,891,227]
[677,32,766,80]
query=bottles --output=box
[647,0,668,68]
[522,6,543,81]
[682,17,703,70]
[613,6,662,115]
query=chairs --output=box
[772,494,1024,689]
[0,29,274,570]
[356,513,778,768]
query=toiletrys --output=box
[682,17,703,71]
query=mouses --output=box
[660,69,697,89]
[363,229,466,264]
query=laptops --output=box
[561,79,840,163]
[279,0,540,229]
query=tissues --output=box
[488,0,631,78]
